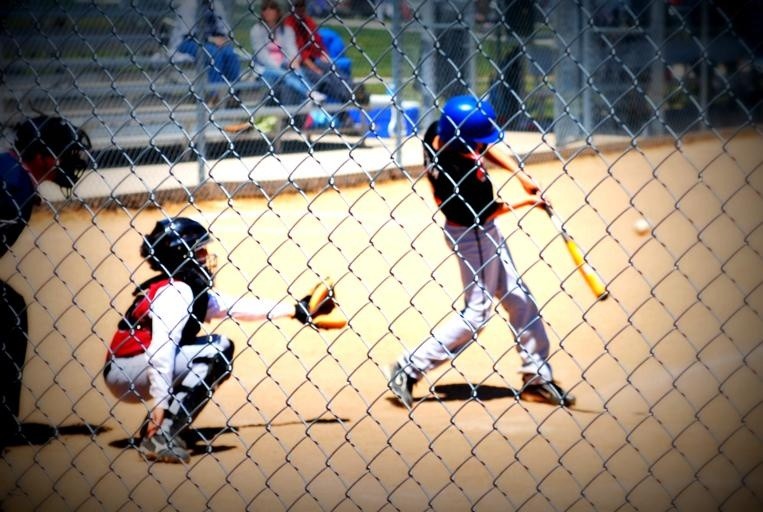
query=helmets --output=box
[139,216,218,285]
[438,94,501,144]
[17,115,92,199]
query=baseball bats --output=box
[527,172,610,303]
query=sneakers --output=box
[386,362,414,410]
[518,380,576,406]
[354,84,369,107]
[139,432,191,465]
[225,97,240,109]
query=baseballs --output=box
[635,217,651,237]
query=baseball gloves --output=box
[294,278,348,331]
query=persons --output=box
[0,114,93,449]
[282,0,370,106]
[387,95,576,409]
[103,217,349,463]
[248,1,327,108]
[168,0,242,111]
